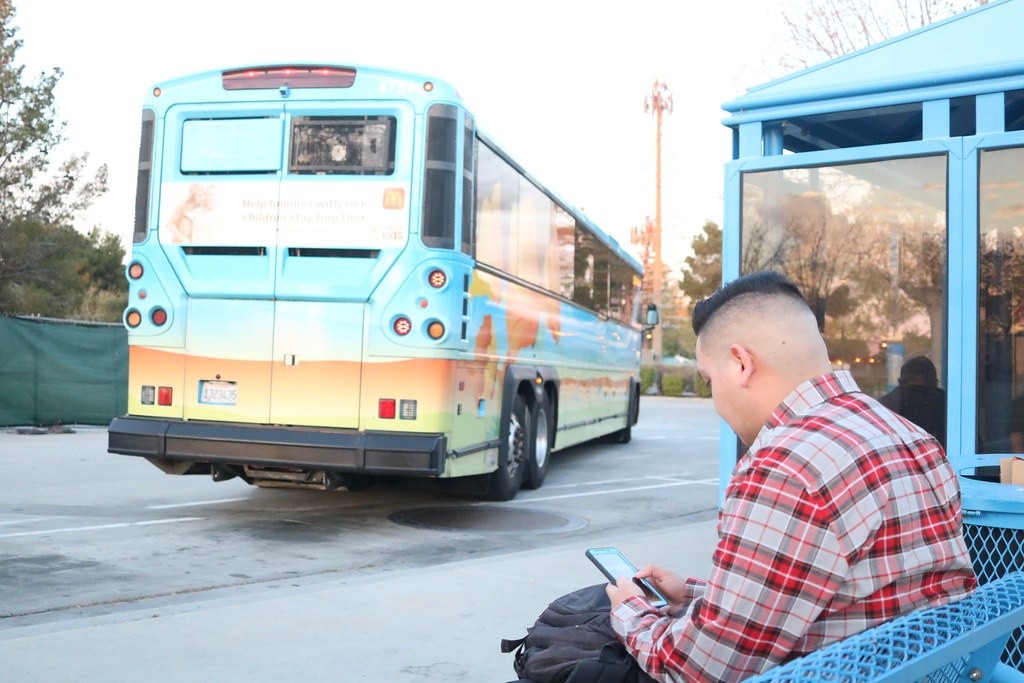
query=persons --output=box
[606,271,978,683]
[876,356,984,455]
[1006,396,1024,453]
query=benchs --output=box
[739,571,1024,683]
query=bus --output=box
[106,63,659,499]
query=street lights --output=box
[642,78,675,392]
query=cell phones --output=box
[585,546,668,609]
